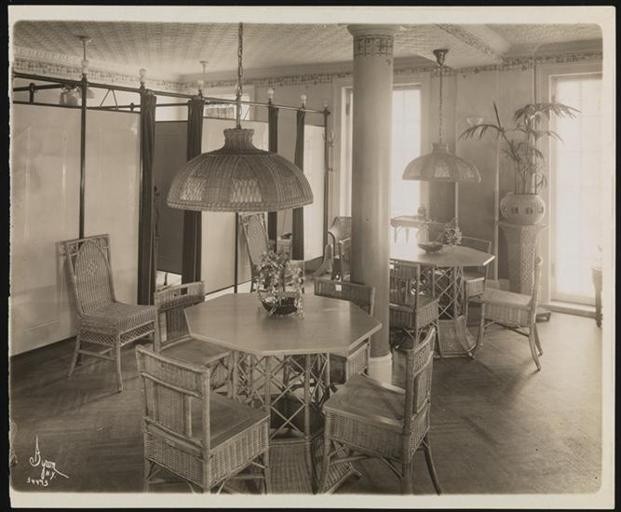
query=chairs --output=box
[340,238,362,280]
[316,325,444,494]
[57,235,162,393]
[241,213,305,294]
[461,236,492,319]
[472,255,546,371]
[328,216,351,276]
[134,344,272,495]
[291,278,375,387]
[154,280,231,399]
[388,262,443,359]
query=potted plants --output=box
[458,99,583,224]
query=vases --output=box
[418,242,445,252]
[260,296,302,318]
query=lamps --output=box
[166,23,315,212]
[74,36,97,99]
[405,46,481,182]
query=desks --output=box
[181,292,384,494]
[391,216,445,244]
[386,241,495,360]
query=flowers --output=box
[250,250,307,312]
[414,216,462,247]
